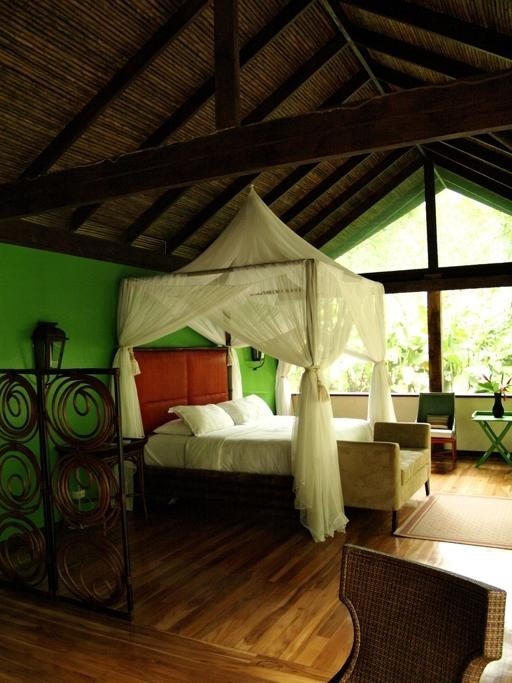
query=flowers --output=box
[475,374,512,395]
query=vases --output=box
[491,395,504,418]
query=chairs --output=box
[328,540,508,683]
[414,393,457,467]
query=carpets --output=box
[391,492,511,550]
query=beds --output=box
[118,347,367,520]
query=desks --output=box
[470,410,512,468]
[56,432,150,535]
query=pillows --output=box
[152,393,276,438]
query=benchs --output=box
[336,422,433,533]
